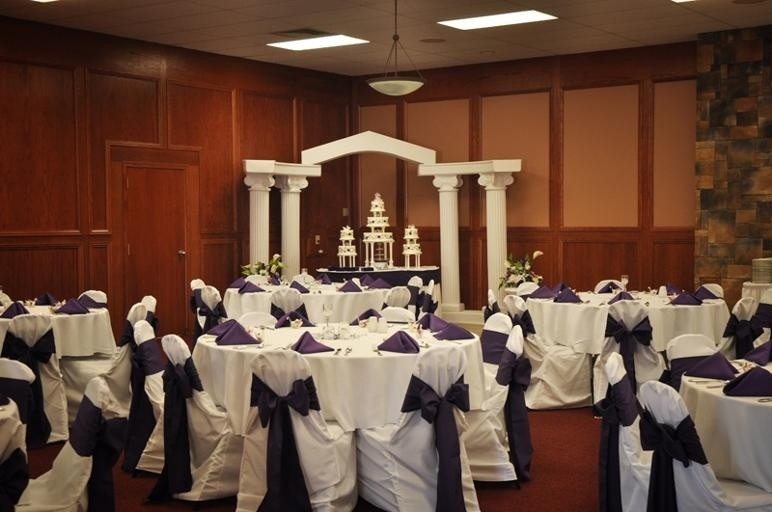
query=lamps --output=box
[369,1,426,99]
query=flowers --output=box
[497,249,545,291]
[240,252,289,283]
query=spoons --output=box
[344,347,352,356]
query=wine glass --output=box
[322,304,335,328]
[621,275,629,293]
[301,267,308,283]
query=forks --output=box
[334,348,341,355]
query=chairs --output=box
[1,275,533,510]
[482,278,757,410]
[600,328,772,512]
